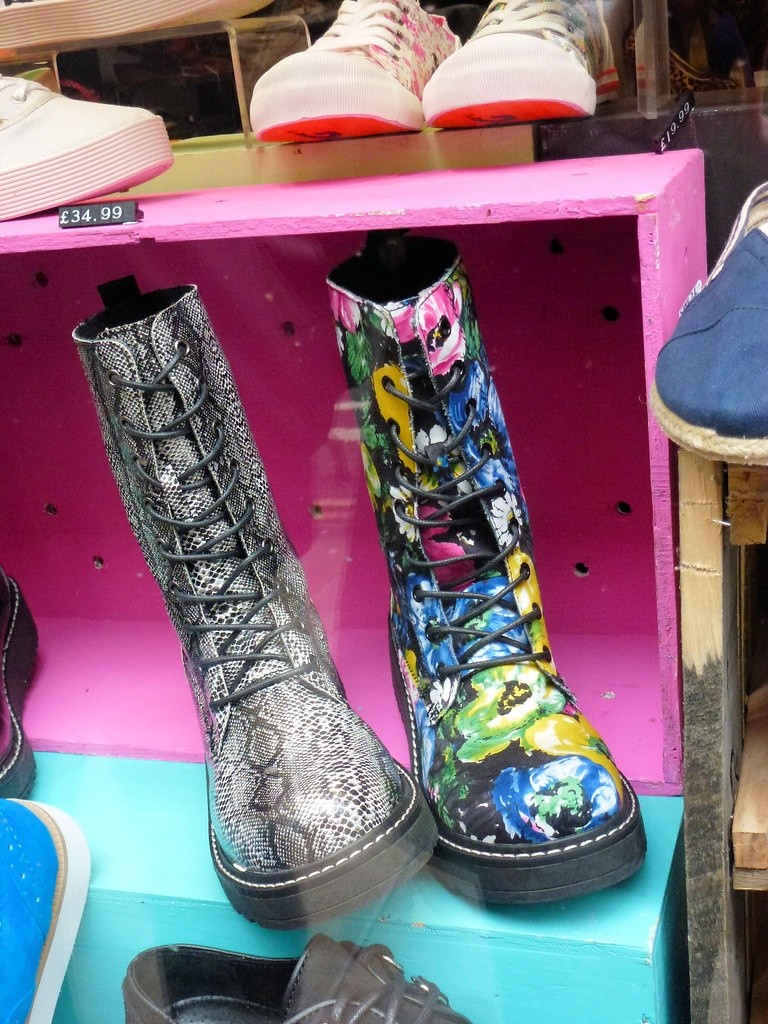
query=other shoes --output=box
[0,799,95,1024]
[648,182,768,462]
[0,572,38,799]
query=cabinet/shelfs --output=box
[0,0,768,1024]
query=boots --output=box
[68,271,438,931]
[322,233,650,902]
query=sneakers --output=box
[1,75,172,221]
[248,0,460,143]
[126,933,471,1024]
[420,0,621,127]
